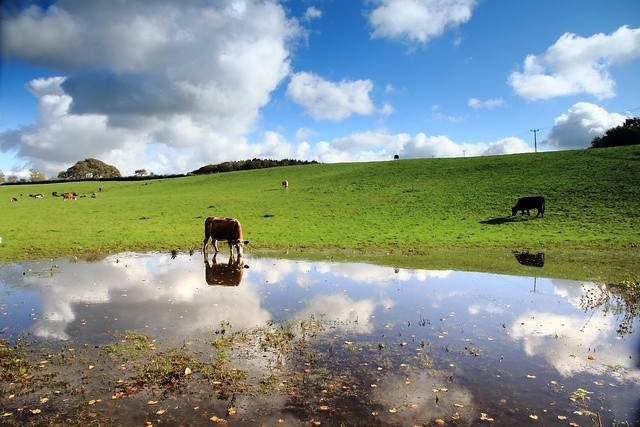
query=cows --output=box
[514,251,545,267]
[282,180,288,189]
[63,193,76,200]
[394,155,399,159]
[203,216,249,257]
[204,257,249,286]
[511,195,545,219]
